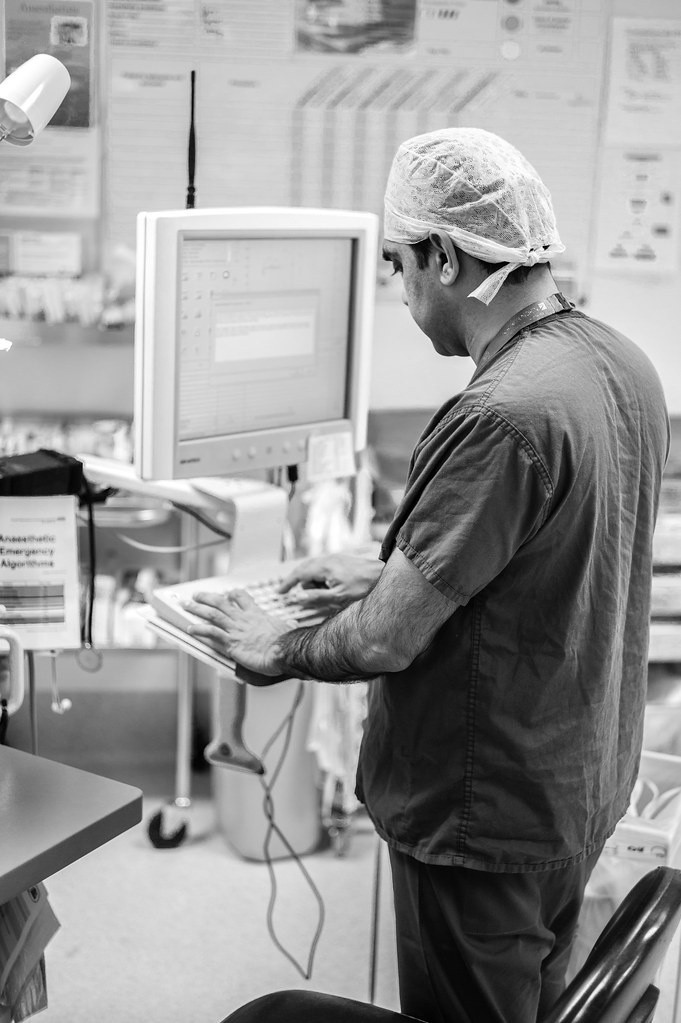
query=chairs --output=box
[220,865,681,1023]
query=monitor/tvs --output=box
[133,207,379,481]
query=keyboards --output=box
[151,539,383,659]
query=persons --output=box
[181,128,672,1023]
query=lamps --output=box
[0,53,70,148]
